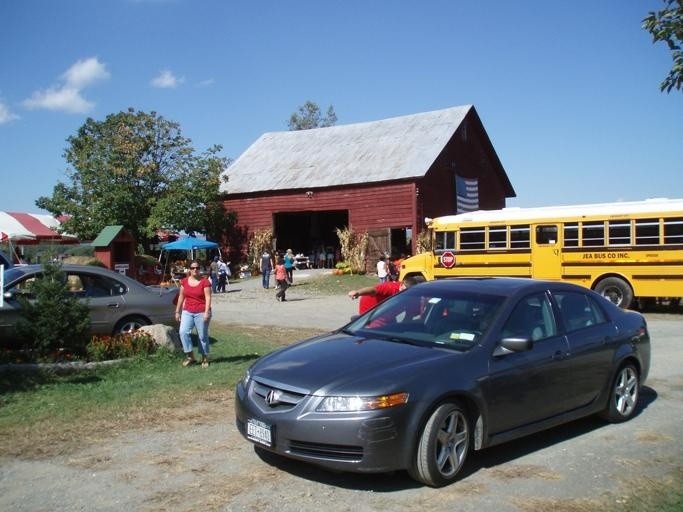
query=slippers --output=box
[202,360,209,368]
[183,359,196,366]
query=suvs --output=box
[0,251,14,273]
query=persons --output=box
[377,254,411,283]
[175,261,212,369]
[207,256,231,294]
[348,274,426,327]
[259,244,343,302]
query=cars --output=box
[233,278,652,487]
[0,262,179,348]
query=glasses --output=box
[190,267,199,270]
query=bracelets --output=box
[175,312,180,313]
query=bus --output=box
[396,198,682,311]
